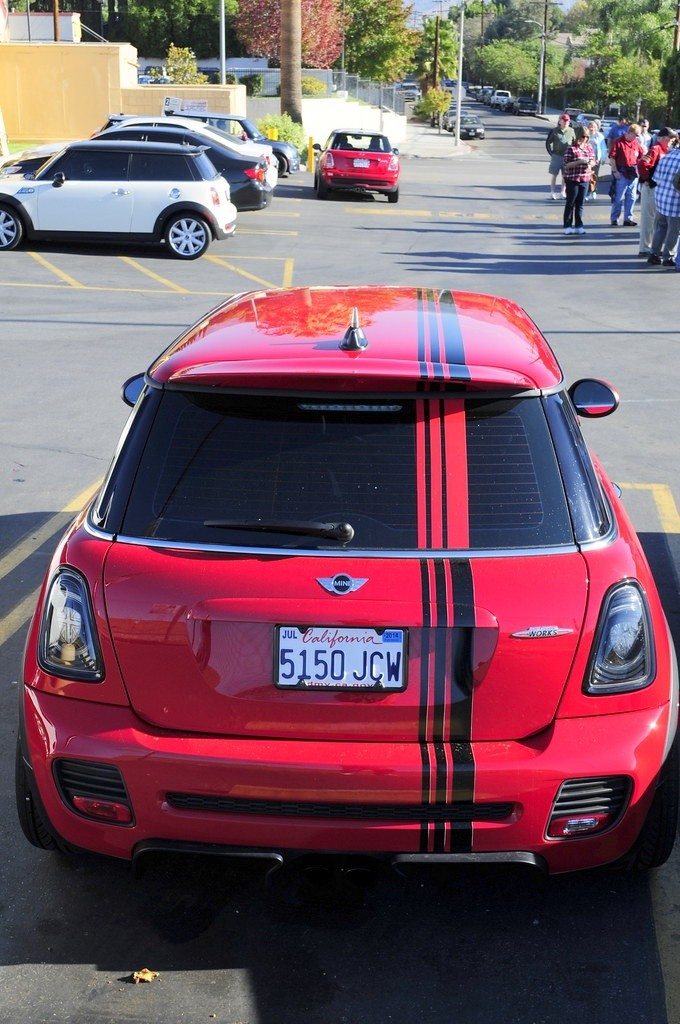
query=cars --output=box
[0,109,300,214]
[599,121,621,143]
[474,87,514,108]
[500,98,517,112]
[312,128,402,205]
[452,116,487,140]
[400,83,418,101]
[436,105,469,132]
[574,114,601,127]
[442,79,469,93]
[558,108,585,128]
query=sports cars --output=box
[15,287,680,906]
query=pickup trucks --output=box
[513,97,538,117]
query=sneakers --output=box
[611,220,617,225]
[550,193,557,200]
[647,254,661,265]
[559,193,566,199]
[623,220,637,226]
[564,228,586,234]
[638,251,663,258]
[663,258,676,266]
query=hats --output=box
[560,114,569,121]
[658,127,678,137]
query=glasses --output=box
[581,136,589,138]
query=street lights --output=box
[523,19,546,113]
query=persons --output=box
[586,116,680,271]
[562,126,596,234]
[546,114,576,200]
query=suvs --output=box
[0,141,241,261]
